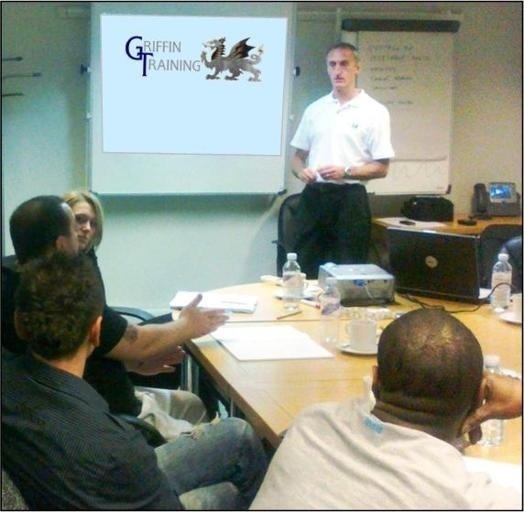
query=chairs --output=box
[0,254,156,365]
[476,223,522,296]
[272,194,302,280]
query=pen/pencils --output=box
[277,310,301,320]
[313,168,319,173]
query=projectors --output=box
[318,261,394,305]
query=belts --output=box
[305,182,365,194]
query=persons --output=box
[243,306,522,511]
[0,246,268,510]
[7,193,229,437]
[287,43,396,280]
[59,183,183,381]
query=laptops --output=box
[387,225,495,304]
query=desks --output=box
[170,283,521,489]
[370,214,522,274]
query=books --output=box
[168,287,258,316]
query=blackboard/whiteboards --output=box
[336,10,449,195]
[91,3,293,197]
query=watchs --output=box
[344,165,353,180]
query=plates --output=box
[498,312,522,326]
[275,291,314,300]
[341,345,379,357]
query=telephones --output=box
[474,181,519,217]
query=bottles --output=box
[318,278,339,347]
[490,253,512,309]
[475,355,504,450]
[282,253,302,314]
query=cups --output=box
[347,318,376,349]
